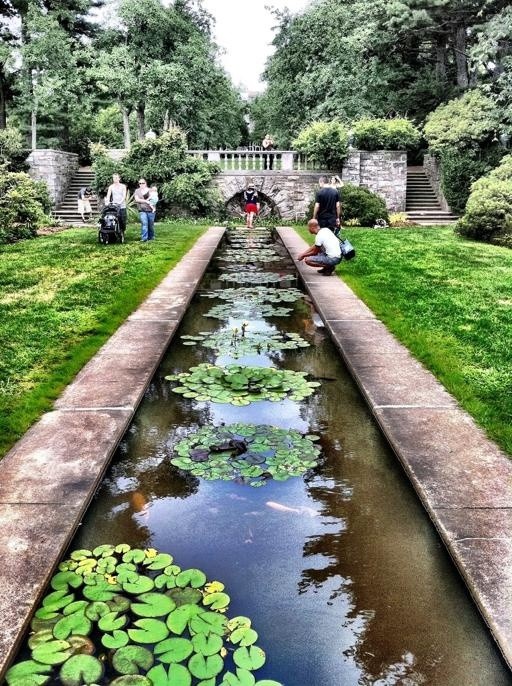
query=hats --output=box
[248,183,256,189]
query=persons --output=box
[243,182,262,230]
[77,187,94,223]
[104,212,118,229]
[262,134,273,170]
[103,173,127,241]
[297,219,342,277]
[134,179,155,243]
[311,174,346,234]
[145,187,159,213]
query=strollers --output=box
[98,201,125,245]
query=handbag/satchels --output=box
[256,202,260,211]
[340,239,355,260]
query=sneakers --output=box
[318,264,335,276]
